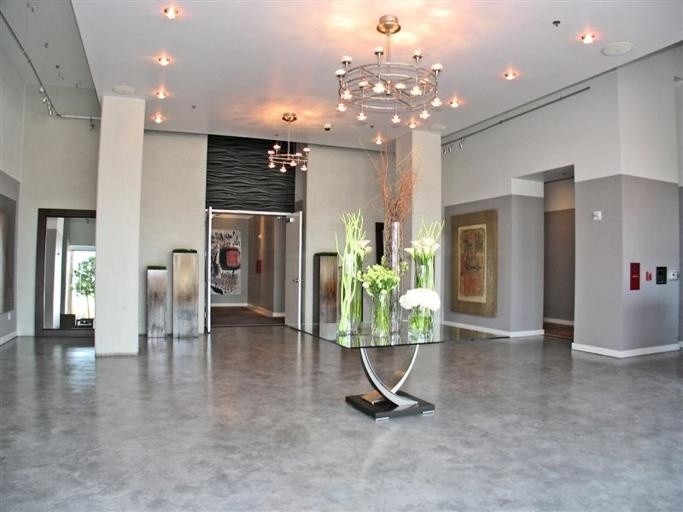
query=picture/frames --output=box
[457,224,487,303]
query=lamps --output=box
[38,85,53,119]
[267,113,310,174]
[334,15,442,145]
[442,137,464,155]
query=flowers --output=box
[335,209,445,311]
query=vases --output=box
[371,292,392,337]
[381,220,402,331]
[407,306,434,341]
[335,255,363,335]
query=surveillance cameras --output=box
[324,123,331,131]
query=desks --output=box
[287,320,510,420]
